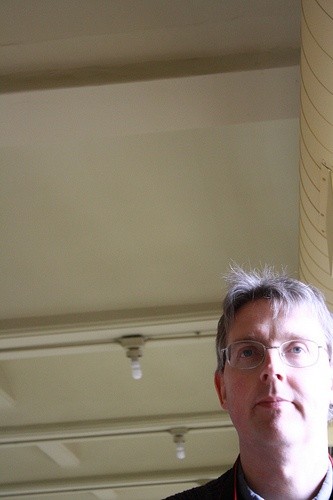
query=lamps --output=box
[117,337,147,380]
[167,426,190,460]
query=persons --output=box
[162,272,332,500]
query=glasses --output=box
[220,339,330,370]
